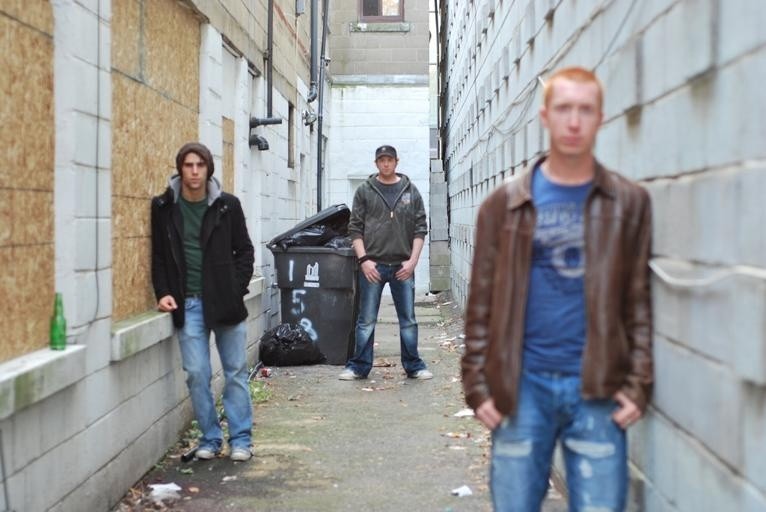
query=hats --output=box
[375,146,398,161]
[175,143,215,178]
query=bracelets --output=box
[356,255,370,264]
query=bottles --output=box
[48,292,67,351]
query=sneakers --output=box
[230,448,251,460]
[407,369,434,380]
[193,449,216,459]
[337,367,360,380]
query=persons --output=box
[150,141,257,461]
[459,65,656,511]
[336,145,437,381]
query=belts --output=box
[184,293,204,298]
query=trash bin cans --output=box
[265,204,356,367]
